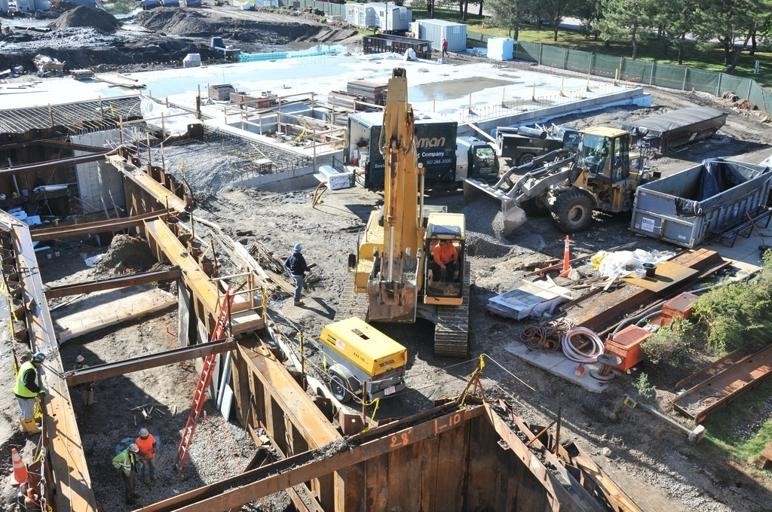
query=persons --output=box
[283,244,310,306]
[112,428,156,505]
[13,350,46,423]
[754,60,760,74]
[442,38,449,58]
[431,239,458,282]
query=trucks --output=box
[343,108,502,198]
[494,122,583,170]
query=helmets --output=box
[294,243,302,250]
[31,353,45,362]
[75,355,85,363]
[129,427,150,452]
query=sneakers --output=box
[20,423,42,436]
[294,302,303,306]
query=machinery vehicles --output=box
[459,121,663,236]
[329,64,474,362]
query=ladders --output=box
[174,284,237,476]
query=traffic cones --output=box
[9,447,29,486]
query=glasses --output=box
[439,243,445,246]
